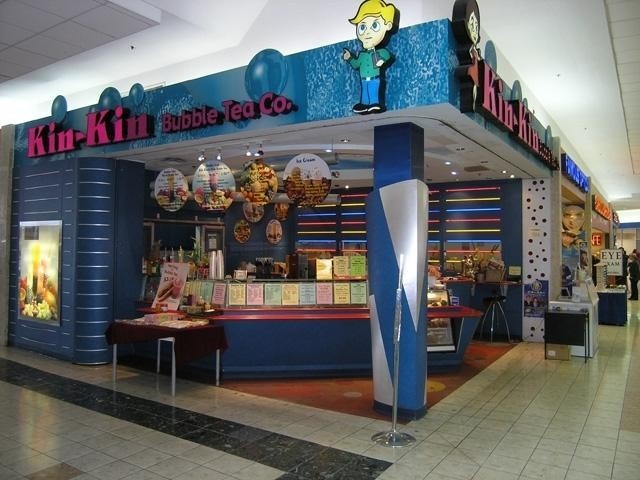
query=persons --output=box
[628,253,640,300]
[618,246,629,291]
[633,248,640,258]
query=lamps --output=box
[198,144,265,161]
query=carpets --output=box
[117,338,520,425]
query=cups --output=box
[208,250,224,281]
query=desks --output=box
[104,322,229,396]
[549,301,599,358]
[597,291,627,325]
[544,309,590,363]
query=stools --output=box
[480,296,511,345]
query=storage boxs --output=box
[546,343,572,360]
